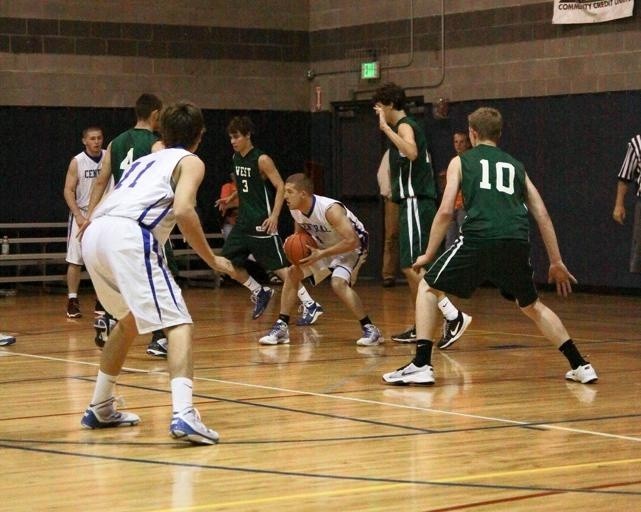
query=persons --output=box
[613,133,640,275]
[382,106,600,386]
[259,172,387,347]
[375,142,402,287]
[444,129,476,253]
[372,81,473,347]
[78,100,236,445]
[214,117,327,326]
[76,94,185,359]
[220,168,243,287]
[62,123,118,317]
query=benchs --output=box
[0,221,225,291]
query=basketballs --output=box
[285,232,317,264]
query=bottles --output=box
[2,235,10,254]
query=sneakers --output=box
[0,297,220,447]
